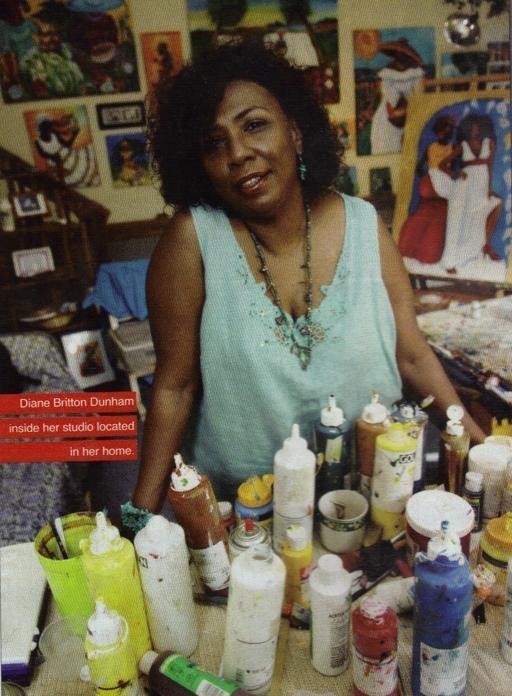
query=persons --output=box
[357,35,429,154]
[437,109,504,273]
[120,46,485,535]
[397,113,453,264]
[1,0,180,193]
[439,51,486,90]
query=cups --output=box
[318,490,367,554]
[40,616,93,680]
[34,511,113,640]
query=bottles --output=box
[476,516,512,607]
[223,544,287,695]
[308,554,351,677]
[168,453,233,594]
[217,500,232,529]
[82,513,152,662]
[440,404,469,497]
[500,561,512,666]
[369,423,417,541]
[281,525,315,605]
[134,514,199,658]
[396,396,429,491]
[499,461,511,516]
[273,424,317,551]
[139,649,240,696]
[470,300,480,320]
[85,596,138,696]
[353,394,393,495]
[469,562,496,611]
[314,395,354,487]
[235,471,273,531]
[350,594,399,696]
[411,519,473,696]
[462,472,483,532]
[376,577,417,614]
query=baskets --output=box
[21,308,79,330]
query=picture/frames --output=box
[12,246,56,278]
[8,190,51,223]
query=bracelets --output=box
[121,497,153,532]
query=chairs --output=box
[97,259,157,422]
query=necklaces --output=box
[238,206,328,374]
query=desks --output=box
[414,287,511,434]
[25,483,512,695]
